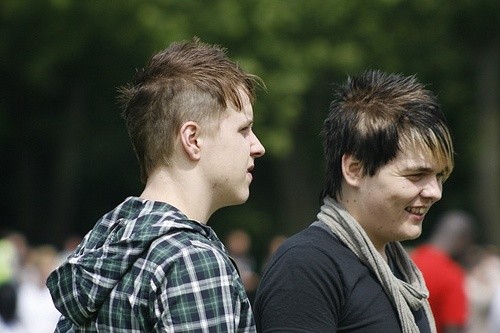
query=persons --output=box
[45,33,267,333]
[2,211,499,333]
[253,66,456,333]
